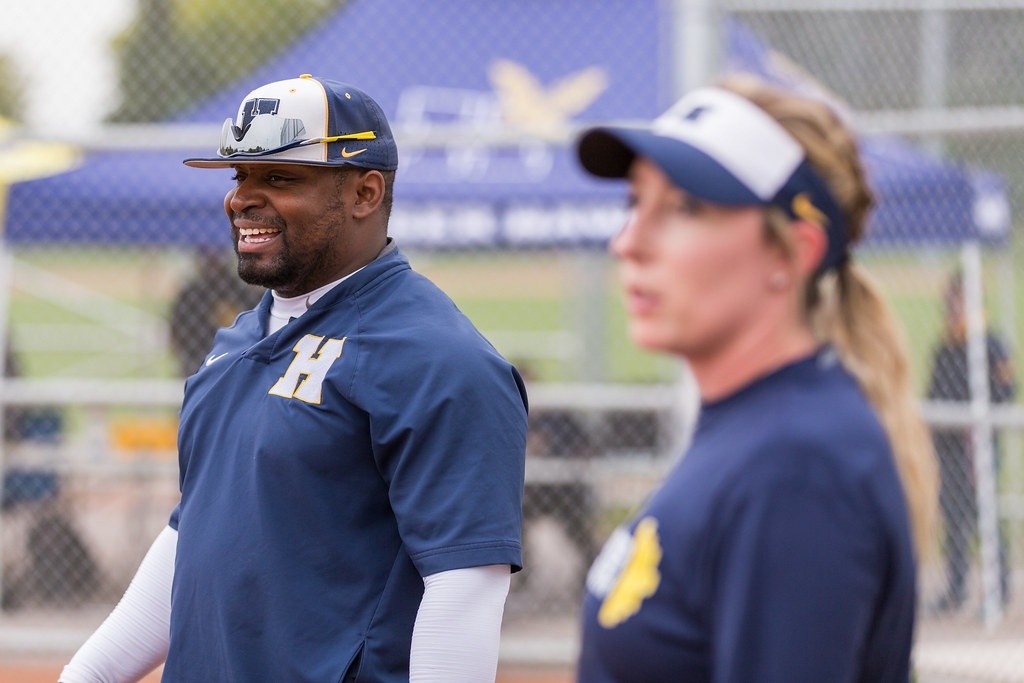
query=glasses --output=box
[217,113,383,158]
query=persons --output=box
[0,241,269,610]
[508,359,603,596]
[59,71,533,683]
[921,270,1019,621]
[572,79,937,683]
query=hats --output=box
[578,85,848,272]
[182,73,398,172]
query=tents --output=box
[0,0,1010,633]
